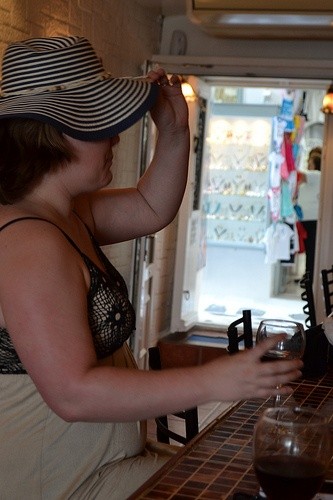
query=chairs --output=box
[149,265,333,446]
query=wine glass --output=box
[255,318,307,406]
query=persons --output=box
[293,146,322,282]
[0,31,302,500]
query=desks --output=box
[127,364,333,500]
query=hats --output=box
[0,34,158,143]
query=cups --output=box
[251,407,333,500]
